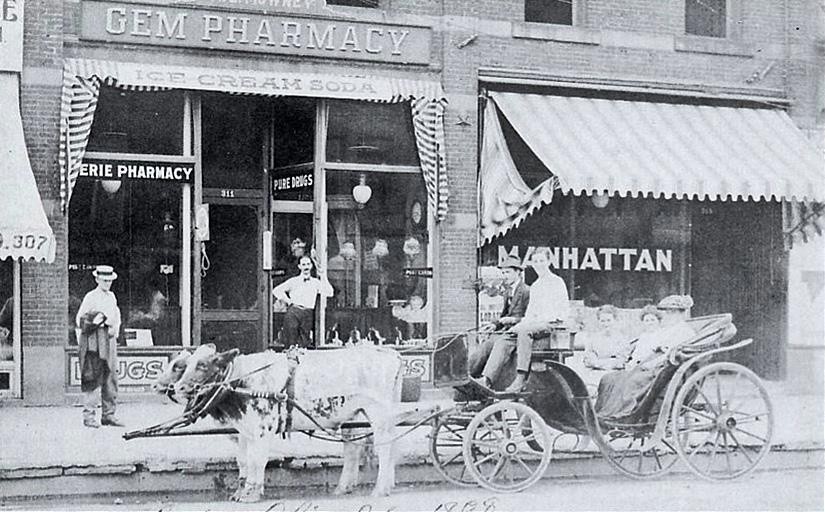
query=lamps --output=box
[339,242,357,310]
[101,180,121,193]
[371,240,390,307]
[591,192,609,208]
[402,237,421,269]
[353,174,372,209]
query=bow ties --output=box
[303,276,310,282]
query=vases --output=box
[402,373,422,401]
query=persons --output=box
[75,265,126,429]
[272,256,335,348]
[578,295,695,419]
[468,247,569,393]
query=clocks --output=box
[410,199,425,228]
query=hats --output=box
[656,294,694,311]
[92,265,118,280]
[497,255,523,272]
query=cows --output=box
[173,344,404,504]
[150,347,375,495]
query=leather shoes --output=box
[83,415,126,428]
[505,380,528,392]
[473,376,490,388]
